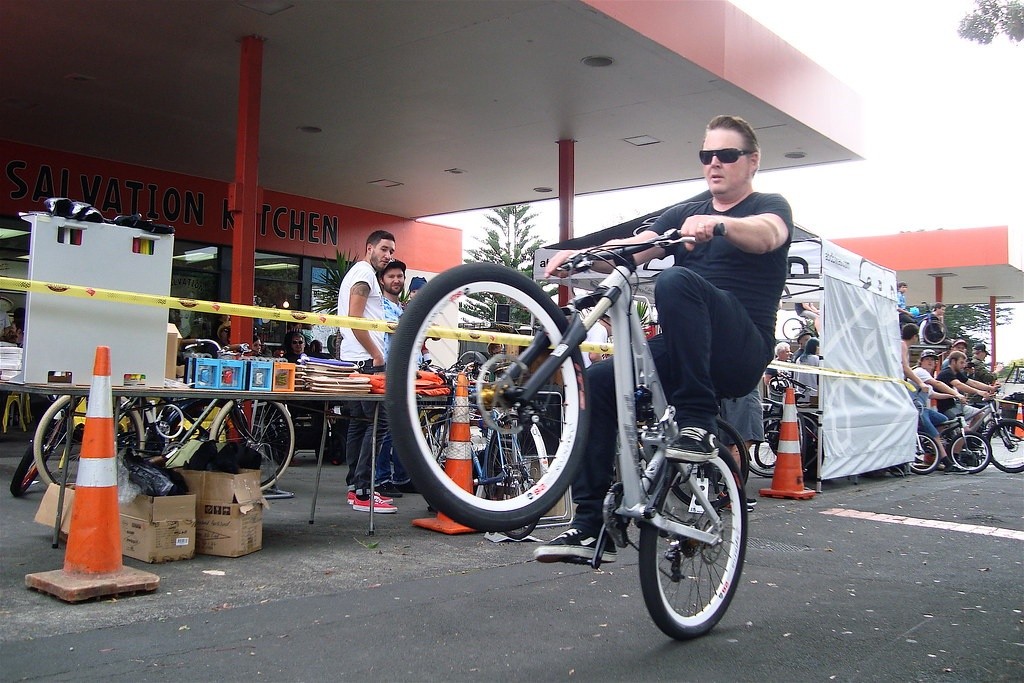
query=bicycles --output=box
[385,220,750,643]
[416,351,547,540]
[749,372,1023,477]
[10,338,296,492]
[902,301,947,345]
[781,318,821,339]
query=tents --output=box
[531,184,919,494]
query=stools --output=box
[3,390,32,433]
[59,396,128,469]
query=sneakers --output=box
[944,464,970,474]
[375,483,403,497]
[962,441,984,450]
[399,482,422,493]
[533,526,617,564]
[665,425,719,461]
[722,492,757,513]
[346,487,398,513]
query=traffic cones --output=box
[759,386,817,501]
[412,375,483,536]
[24,344,162,605]
[1013,404,1024,438]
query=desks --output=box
[0,381,448,548]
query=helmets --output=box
[378,256,407,277]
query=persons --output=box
[900,324,928,393]
[571,308,616,368]
[968,343,997,386]
[283,333,306,364]
[897,281,917,323]
[214,315,230,344]
[791,330,819,402]
[534,117,794,564]
[288,321,323,353]
[795,303,820,337]
[400,277,440,364]
[930,302,954,345]
[253,335,261,354]
[376,255,422,497]
[762,342,790,403]
[906,349,970,475]
[338,230,398,514]
[10,322,24,348]
[934,351,986,462]
[940,338,997,398]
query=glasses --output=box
[291,340,304,345]
[699,148,757,165]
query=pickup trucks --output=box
[999,362,1024,416]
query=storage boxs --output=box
[165,324,183,380]
[34,467,270,563]
[184,357,295,392]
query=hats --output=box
[410,277,428,290]
[952,339,968,347]
[974,344,992,356]
[797,330,813,344]
[921,349,939,360]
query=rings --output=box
[697,223,705,229]
